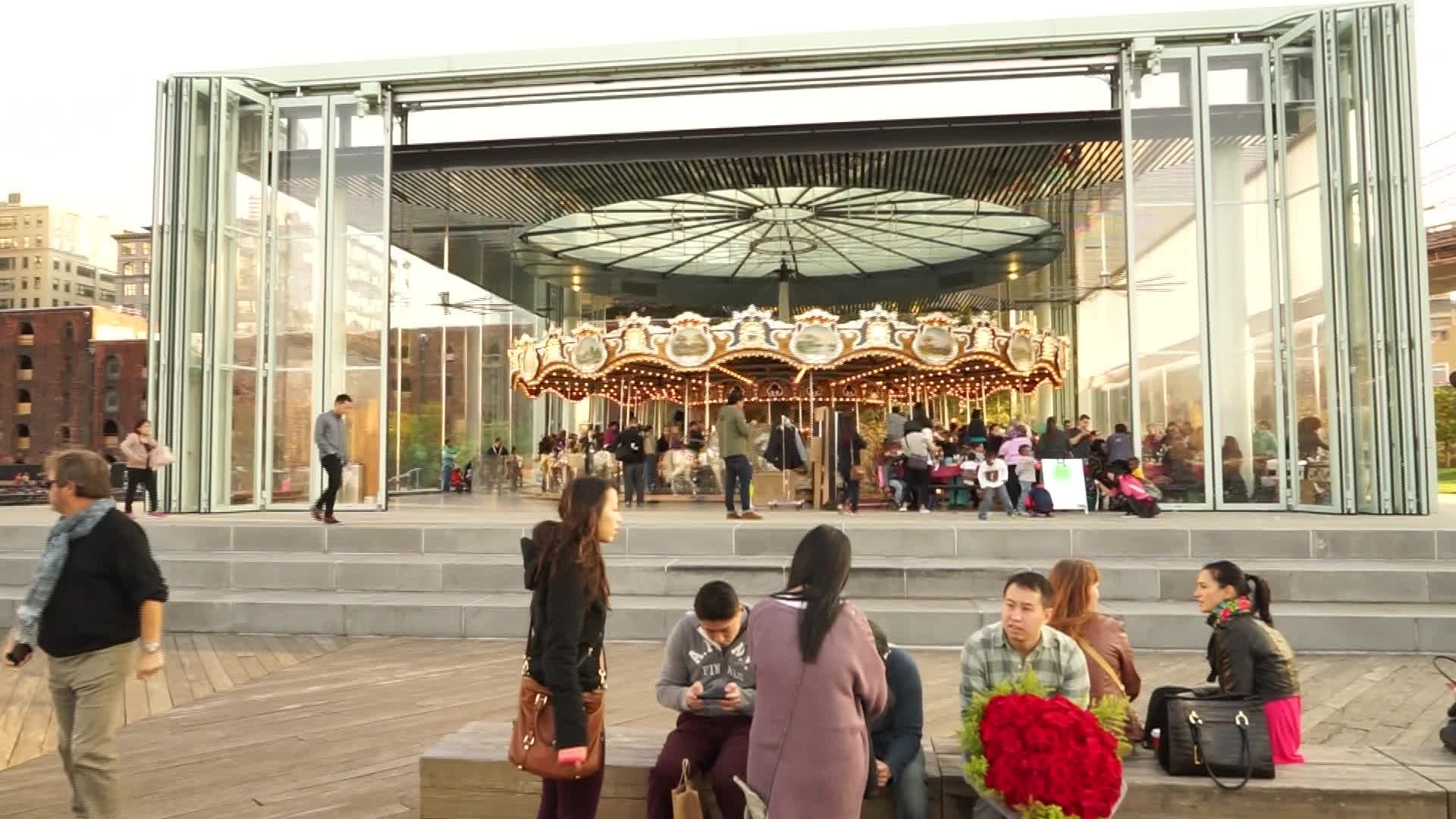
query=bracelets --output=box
[140,643,162,654]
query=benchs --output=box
[418,721,944,819]
[928,484,981,508]
[1371,745,1456,819]
[929,735,1449,818]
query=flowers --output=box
[956,670,1144,819]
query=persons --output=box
[646,579,756,819]
[856,620,929,819]
[1144,563,1303,765]
[1043,558,1147,757]
[442,381,1336,523]
[520,477,621,819]
[120,418,170,520]
[0,448,169,819]
[746,522,887,819]
[308,393,355,526]
[960,570,1091,819]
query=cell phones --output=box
[5,642,33,665]
[696,690,729,701]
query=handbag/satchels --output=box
[1124,702,1143,741]
[615,431,631,462]
[1159,699,1274,788]
[672,758,703,819]
[850,465,865,480]
[1144,482,1162,500]
[907,453,928,470]
[734,775,767,819]
[152,446,172,467]
[508,677,604,779]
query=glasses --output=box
[44,480,58,490]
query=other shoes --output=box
[726,511,741,519]
[324,513,339,523]
[311,509,323,521]
[920,506,930,514]
[899,502,910,512]
[625,501,632,508]
[978,513,988,520]
[638,503,644,508]
[742,511,763,520]
[1008,511,1015,518]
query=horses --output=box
[553,450,724,497]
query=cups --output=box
[1151,728,1161,761]
